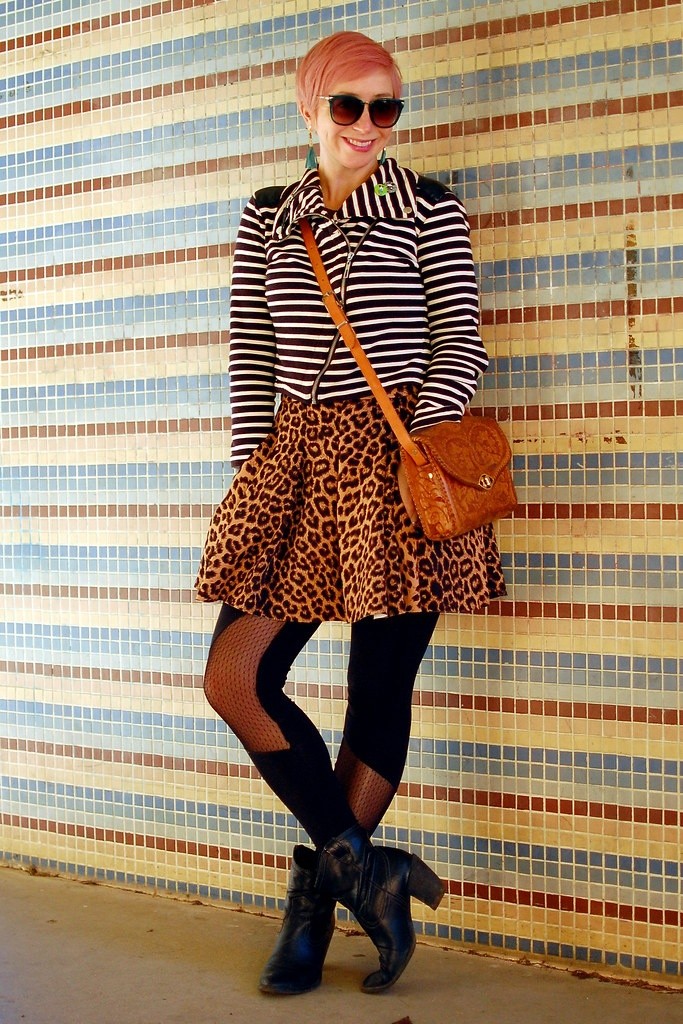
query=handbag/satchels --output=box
[400,416,520,541]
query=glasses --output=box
[318,95,405,128]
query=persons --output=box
[193,34,510,998]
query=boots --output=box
[318,827,446,993]
[258,845,336,997]
